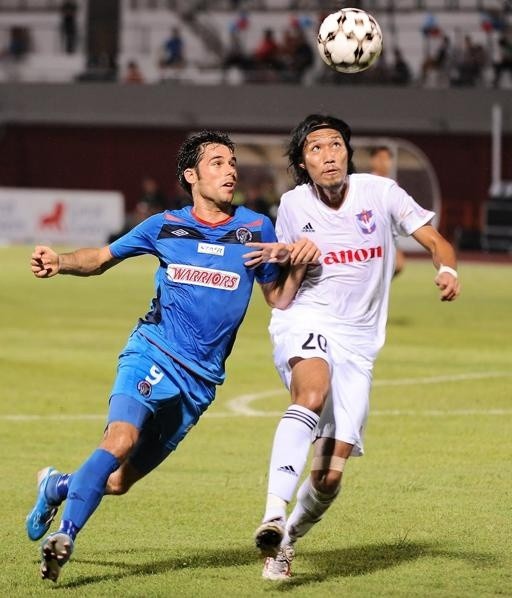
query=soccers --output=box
[318,7,382,75]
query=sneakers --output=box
[25,465,58,541]
[39,530,73,582]
[263,547,294,580]
[253,522,285,558]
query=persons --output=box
[23,129,324,582]
[240,112,462,579]
[126,0,511,275]
